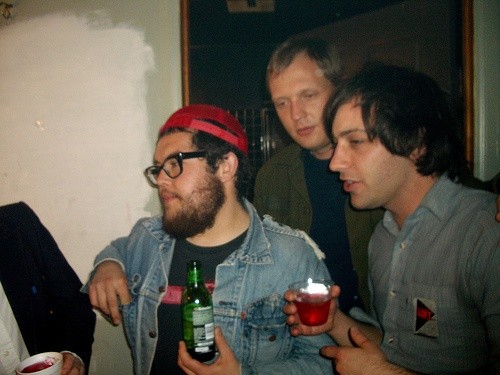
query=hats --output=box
[159,104,248,160]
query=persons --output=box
[281,62,500,375]
[82,104,342,375]
[253,34,388,325]
[0,199,97,375]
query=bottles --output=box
[183,260,216,362]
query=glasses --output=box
[144,151,223,188]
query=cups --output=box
[15,352,63,375]
[288,281,333,326]
